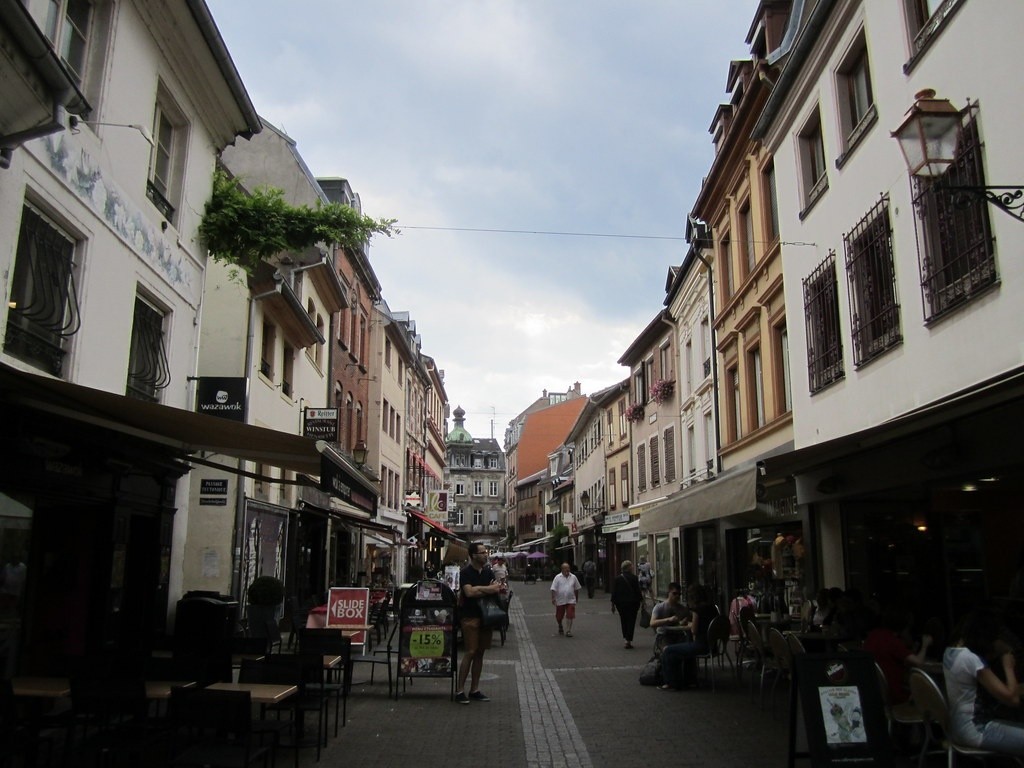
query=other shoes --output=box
[559,625,573,637]
[657,683,696,692]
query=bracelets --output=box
[920,646,928,649]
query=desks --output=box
[664,625,695,644]
[322,654,342,737]
[204,682,297,767]
[9,677,197,767]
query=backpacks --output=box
[638,561,652,589]
[586,561,594,576]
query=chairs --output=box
[699,603,1024,768]
[0,590,401,768]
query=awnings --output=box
[408,451,442,485]
[409,508,470,550]
[638,462,758,535]
[0,0,92,151]
[303,500,403,536]
[249,274,325,350]
[0,360,380,500]
[553,478,573,495]
[546,495,559,505]
[513,534,554,550]
[298,252,348,316]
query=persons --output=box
[582,557,597,599]
[636,555,656,607]
[424,559,438,579]
[455,542,501,704]
[942,603,1024,756]
[649,582,719,691]
[549,563,581,637]
[486,556,511,590]
[863,607,932,755]
[610,560,646,649]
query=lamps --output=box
[892,86,1023,223]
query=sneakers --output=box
[456,690,490,703]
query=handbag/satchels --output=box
[640,606,651,628]
[639,663,662,685]
[732,660,771,699]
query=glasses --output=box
[473,551,488,554]
[669,591,682,595]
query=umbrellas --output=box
[489,551,529,559]
[526,551,550,580]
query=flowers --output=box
[627,404,644,422]
[652,379,675,404]
